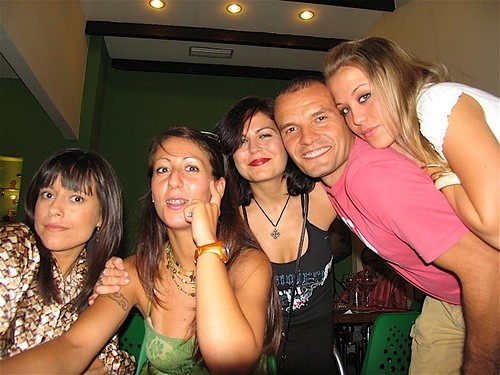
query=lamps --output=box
[9,195,18,205]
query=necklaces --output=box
[253,192,292,239]
[166,242,197,298]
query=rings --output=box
[98,271,106,285]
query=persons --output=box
[214,35,500,375]
[0,148,136,375]
[1,125,281,375]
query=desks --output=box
[332,310,414,375]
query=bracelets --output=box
[436,175,460,190]
[421,160,447,170]
[430,169,452,182]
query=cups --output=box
[349,291,362,309]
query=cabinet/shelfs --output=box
[0,188,19,224]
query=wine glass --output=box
[342,272,361,308]
[355,273,378,307]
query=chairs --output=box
[117,312,145,362]
[360,312,421,375]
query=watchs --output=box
[193,241,230,264]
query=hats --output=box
[361,247,379,263]
